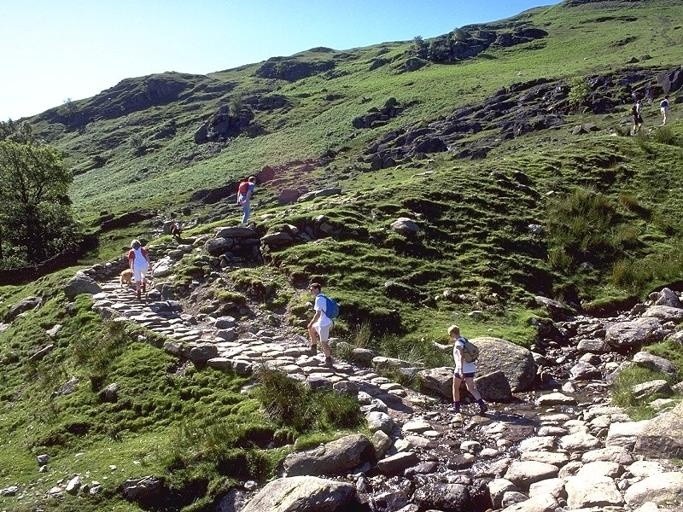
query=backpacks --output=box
[239,182,249,193]
[326,297,340,318]
[463,342,479,363]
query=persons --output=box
[431,325,488,414]
[307,282,340,367]
[632,100,644,134]
[237,176,256,223]
[128,239,152,297]
[660,95,670,125]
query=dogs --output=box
[169,222,182,238]
[119,268,134,291]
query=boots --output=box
[136,283,146,299]
[477,399,487,414]
[317,356,332,367]
[304,344,317,355]
[448,400,460,413]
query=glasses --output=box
[449,335,451,336]
[310,289,312,291]
